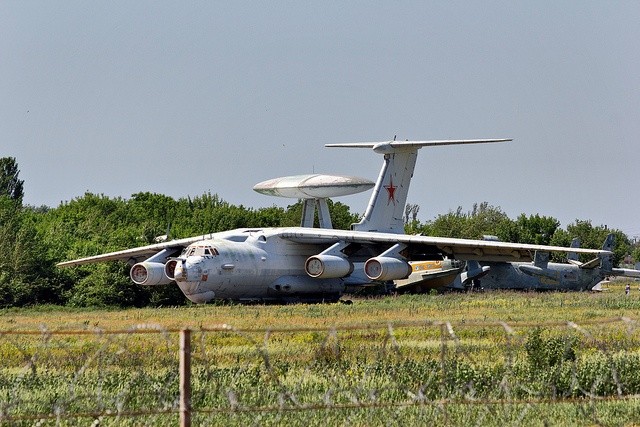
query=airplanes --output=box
[444,232,614,291]
[55,134,614,304]
